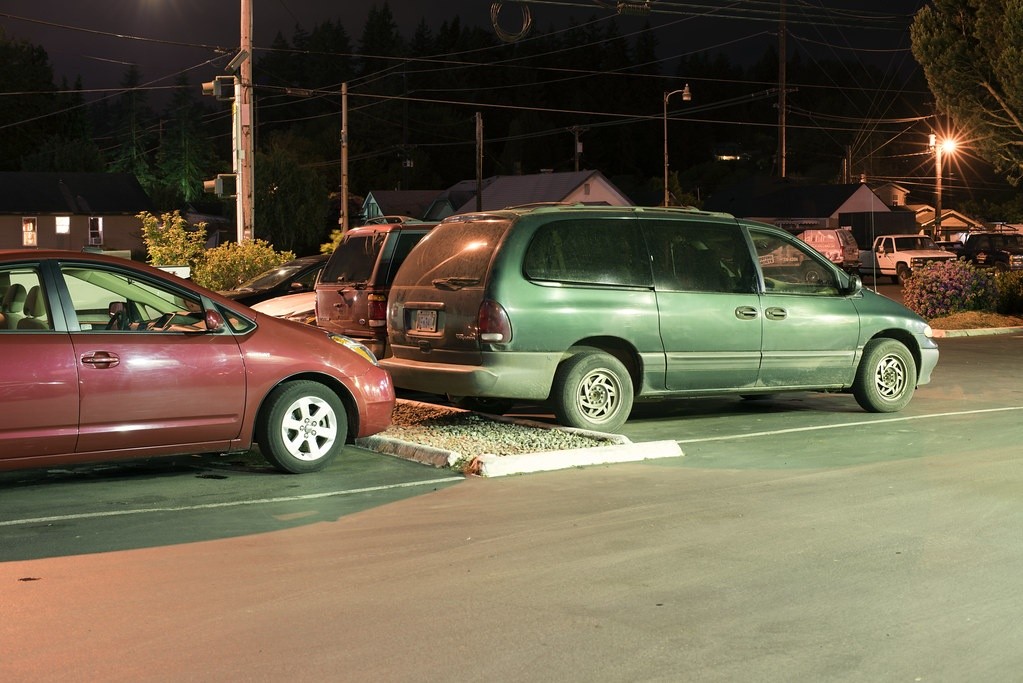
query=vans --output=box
[765,228,860,286]
[378,197,940,436]
[315,216,445,343]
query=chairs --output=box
[695,248,732,290]
[17,285,50,330]
[1,283,28,330]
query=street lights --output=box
[933,136,955,242]
[664,83,693,207]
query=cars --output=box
[0,246,396,475]
[932,241,965,261]
[213,254,330,327]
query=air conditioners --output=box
[90,238,102,245]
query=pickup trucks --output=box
[856,234,958,286]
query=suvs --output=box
[964,229,1023,275]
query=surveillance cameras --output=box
[225,50,249,75]
